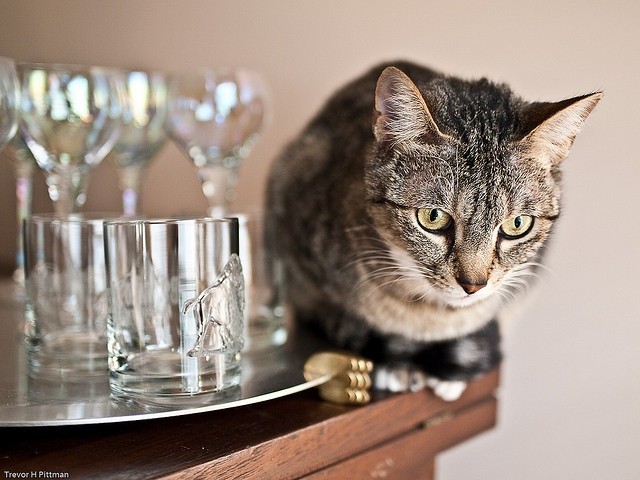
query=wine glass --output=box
[163,70,265,221]
[1,53,20,158]
[14,60,119,221]
[12,64,35,282]
[99,65,176,219]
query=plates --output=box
[1,298,343,428]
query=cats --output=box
[263,55,604,403]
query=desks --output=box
[1,360,501,480]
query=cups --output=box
[23,378,116,429]
[205,205,289,346]
[23,213,134,385]
[103,217,246,395]
[107,389,242,422]
